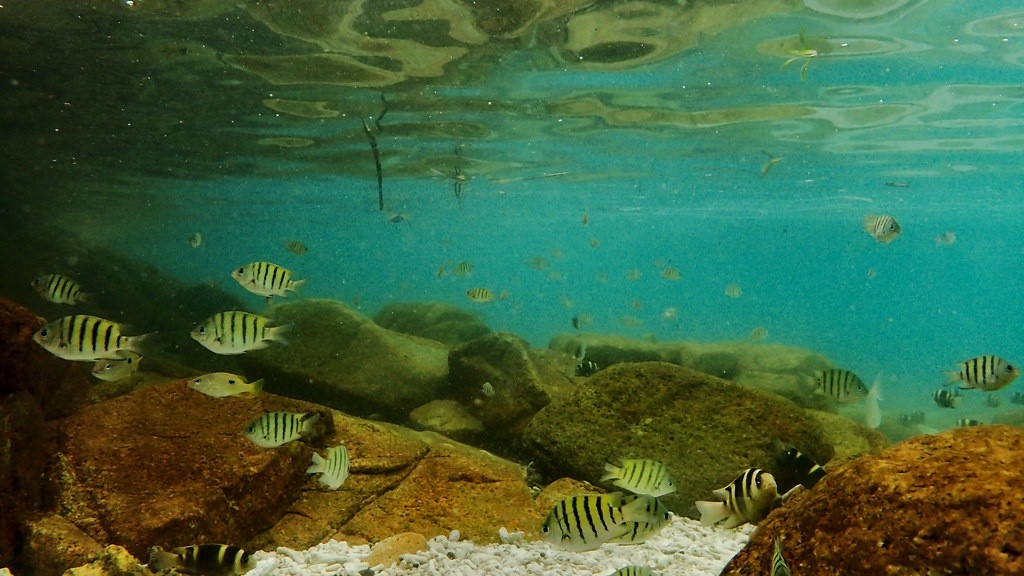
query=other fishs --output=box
[33,154,1023,576]
